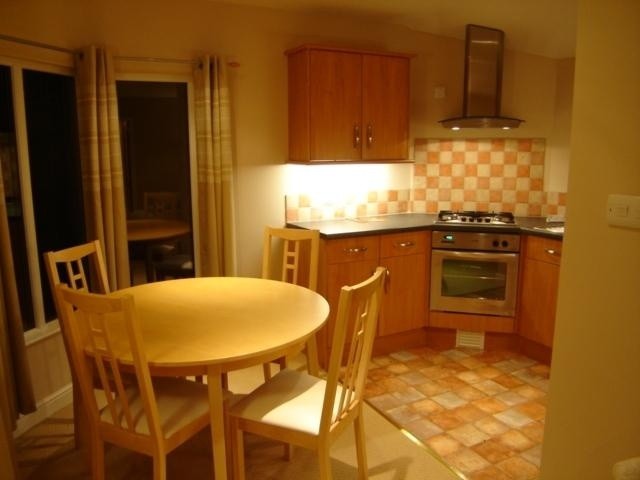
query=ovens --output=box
[430,230,521,317]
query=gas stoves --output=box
[434,210,520,230]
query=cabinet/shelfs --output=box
[284,45,414,164]
[329,229,430,357]
[517,235,561,368]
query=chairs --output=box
[43,239,186,452]
[53,282,233,480]
[261,225,320,383]
[128,190,193,285]
[226,266,387,480]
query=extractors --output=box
[438,24,527,130]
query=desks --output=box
[83,275,330,480]
